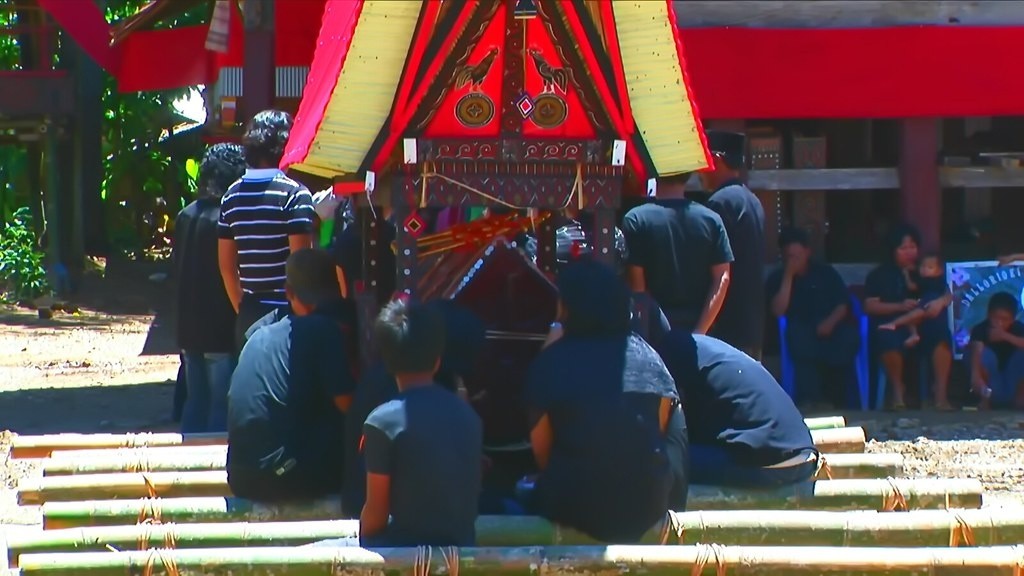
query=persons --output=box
[171,109,955,549]
[997,252,1024,267]
[963,292,1024,412]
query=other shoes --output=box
[933,400,958,411]
[888,401,906,411]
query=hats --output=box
[705,128,747,158]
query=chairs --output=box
[778,295,870,410]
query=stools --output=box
[873,350,928,411]
